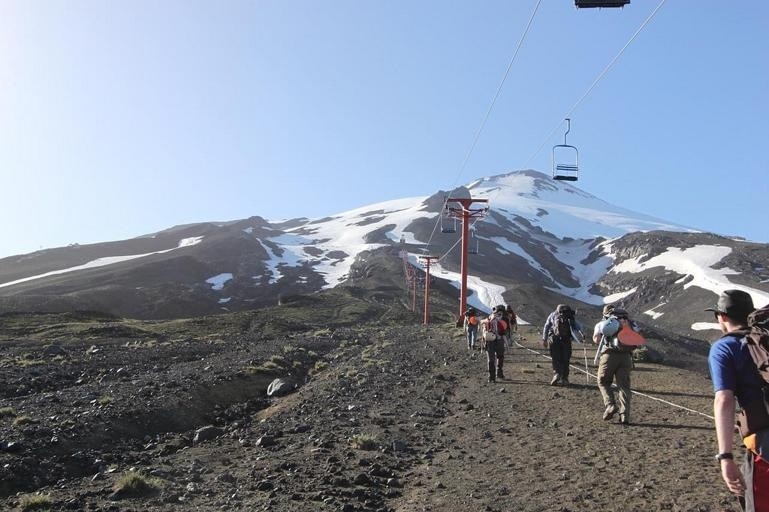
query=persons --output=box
[542,304,586,386]
[480,305,510,382]
[703,290,769,512]
[593,305,635,423]
[505,305,517,347]
[464,307,481,349]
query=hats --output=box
[603,304,614,316]
[704,290,754,322]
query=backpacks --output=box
[552,303,572,339]
[733,304,769,385]
[481,317,499,342]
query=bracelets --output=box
[715,453,734,462]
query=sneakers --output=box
[602,404,628,424]
[551,373,569,386]
